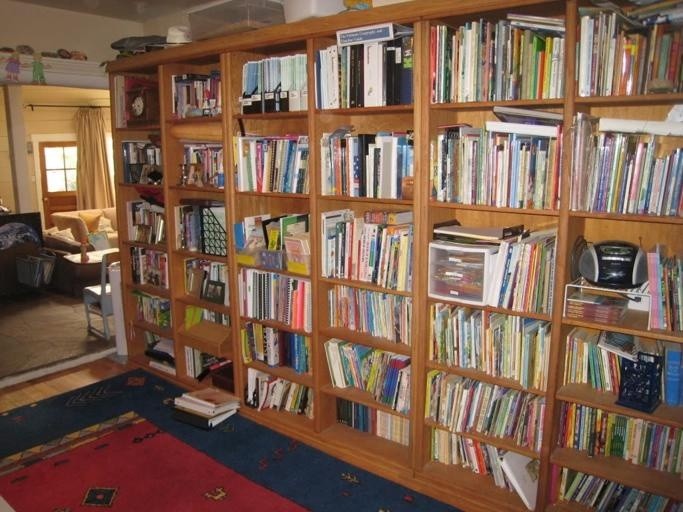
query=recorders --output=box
[580,239,650,288]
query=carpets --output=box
[1,367,460,512]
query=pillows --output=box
[79,212,101,233]
[45,225,75,240]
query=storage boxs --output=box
[186,0,346,41]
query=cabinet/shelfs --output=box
[105,0,683,512]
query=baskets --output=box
[614,351,664,414]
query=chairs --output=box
[83,252,120,348]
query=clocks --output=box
[123,84,154,126]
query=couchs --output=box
[50,207,118,248]
[43,232,97,296]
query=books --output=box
[114,75,177,376]
[428,1,683,216]
[549,243,682,511]
[232,54,314,418]
[172,74,240,431]
[313,22,415,445]
[428,225,557,511]
[18,252,56,287]
